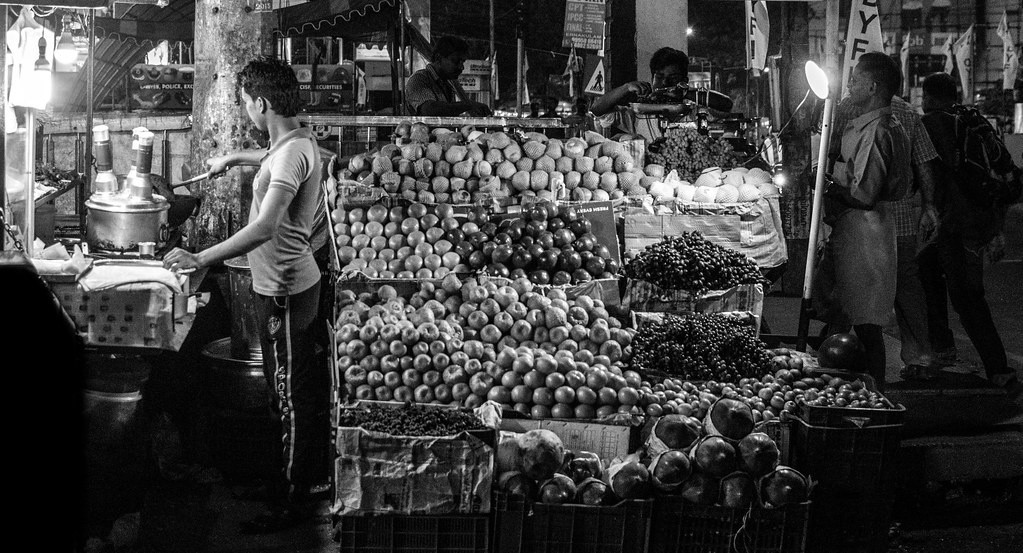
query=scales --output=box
[628,101,692,136]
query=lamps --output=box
[806,59,838,101]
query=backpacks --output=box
[927,105,1023,204]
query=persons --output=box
[816,51,912,395]
[909,70,1016,397]
[828,88,942,365]
[590,45,735,133]
[163,61,328,514]
[402,36,490,118]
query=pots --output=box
[86,192,173,261]
[165,194,202,237]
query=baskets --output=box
[763,368,908,552]
[334,510,492,553]
[491,485,813,553]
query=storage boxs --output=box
[322,165,908,553]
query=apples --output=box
[332,201,641,423]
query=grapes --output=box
[621,127,772,381]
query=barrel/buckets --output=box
[13,203,57,249]
[224,256,267,362]
[201,335,284,497]
